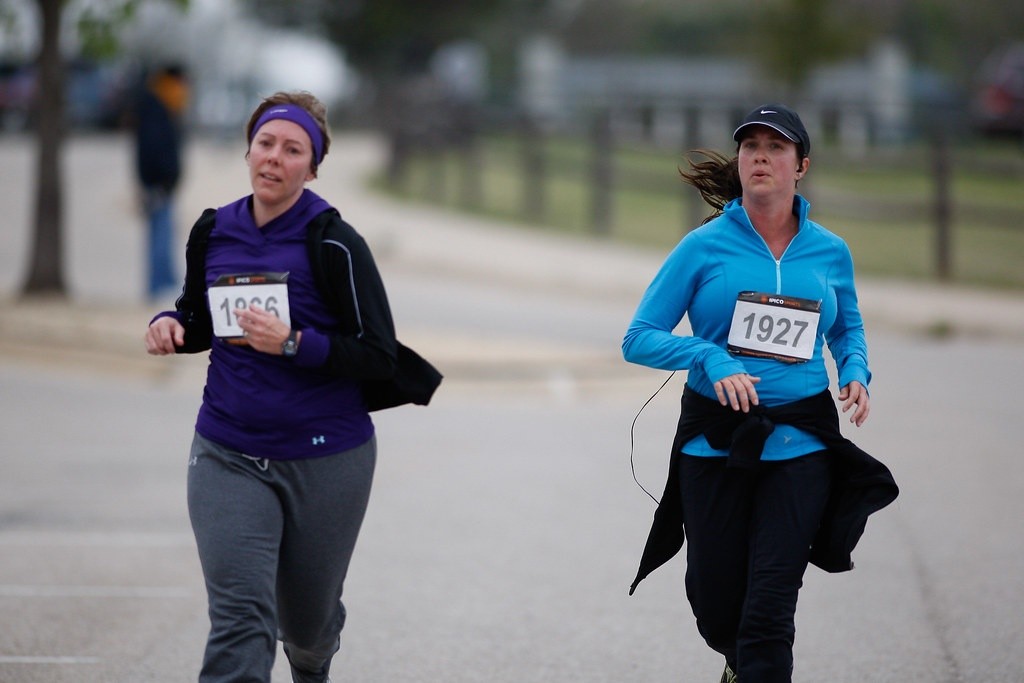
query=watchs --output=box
[282,329,299,357]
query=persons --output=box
[622,106,898,683]
[143,93,444,683]
[127,60,187,303]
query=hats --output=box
[733,103,811,157]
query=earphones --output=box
[796,162,804,172]
